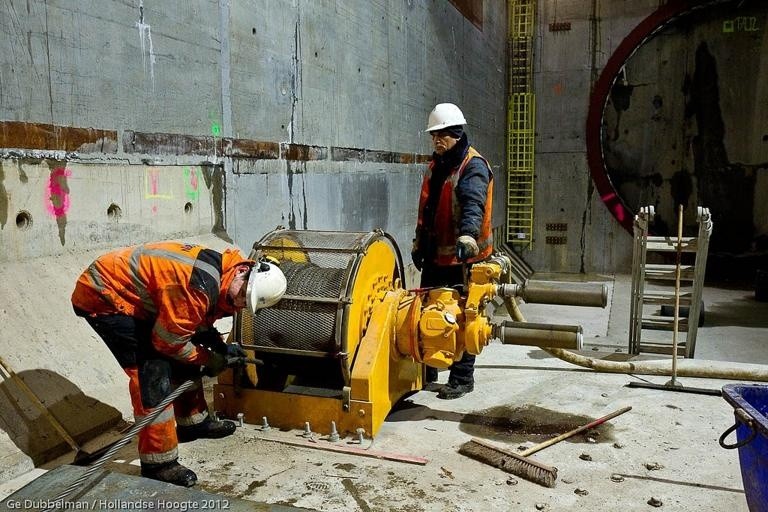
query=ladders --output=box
[629,206,713,360]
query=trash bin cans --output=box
[721,382,768,512]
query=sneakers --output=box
[141,461,197,485]
[176,414,236,443]
[439,379,474,400]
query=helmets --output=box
[246,262,287,314]
[424,102,468,133]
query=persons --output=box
[409,102,496,400]
[68,239,288,486]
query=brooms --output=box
[458,405,632,490]
[0,358,132,467]
[628,204,722,395]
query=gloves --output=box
[197,344,248,377]
[456,235,479,263]
[411,240,425,271]
